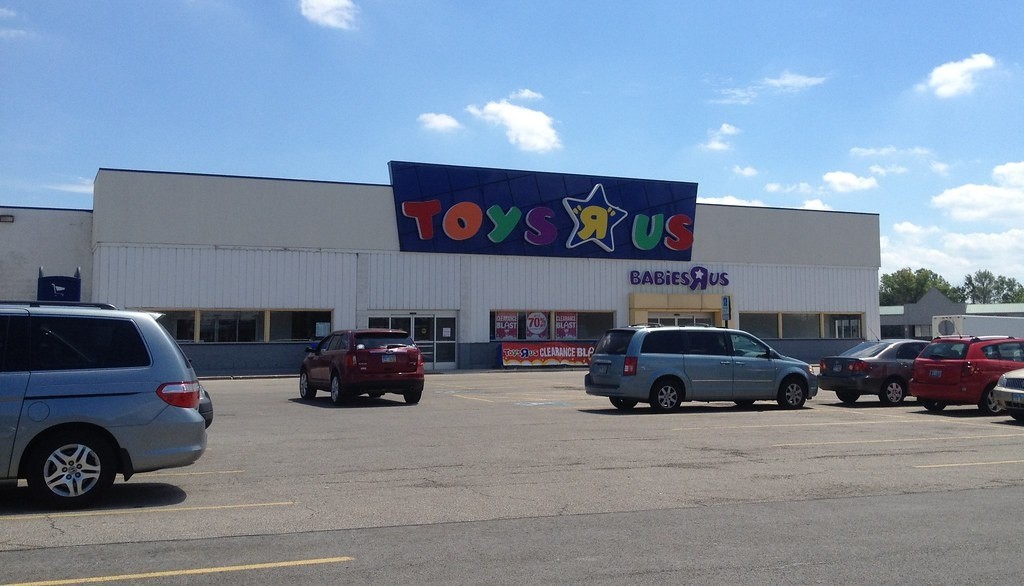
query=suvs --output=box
[908,335,1024,416]
[299,327,425,407]
[584,323,819,414]
[0,300,216,508]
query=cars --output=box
[816,339,931,407]
[994,368,1024,421]
